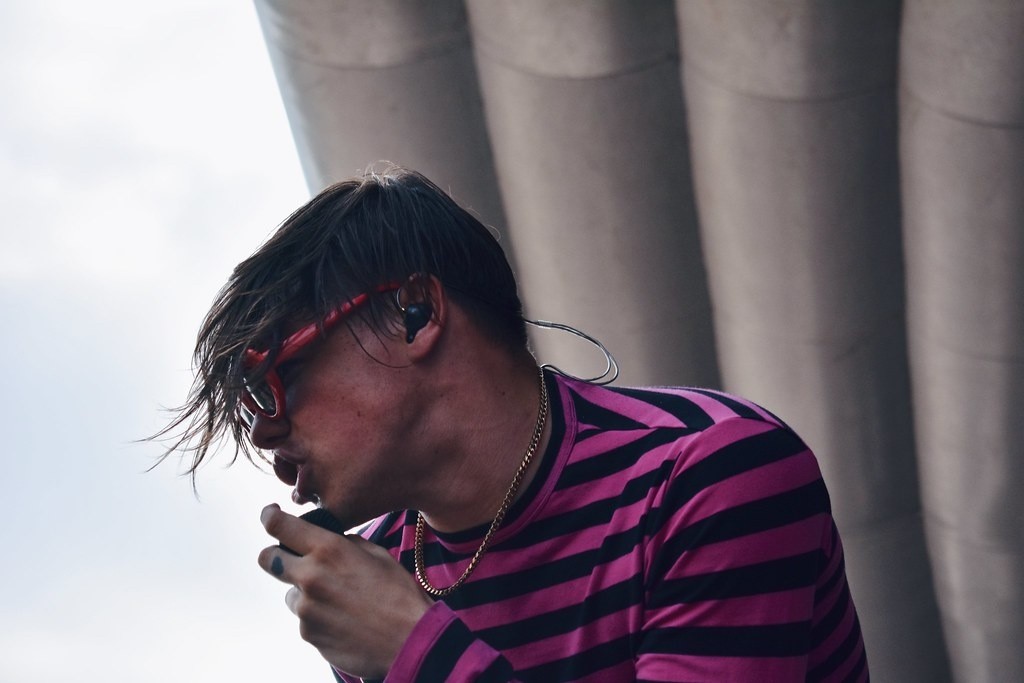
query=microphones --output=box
[277,508,347,683]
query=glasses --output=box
[234,281,402,433]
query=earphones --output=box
[404,299,434,343]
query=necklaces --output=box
[414,367,548,596]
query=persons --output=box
[136,161,874,683]
